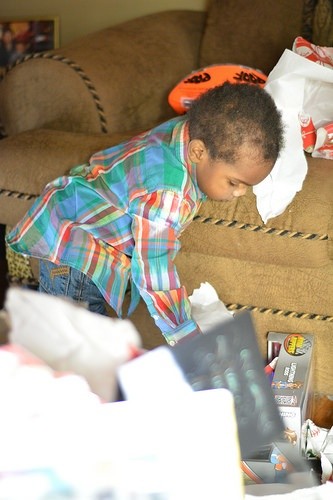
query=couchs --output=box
[0,0,332,397]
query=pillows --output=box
[292,36,333,160]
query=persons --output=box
[5,81,287,349]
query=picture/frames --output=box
[0,15,60,75]
